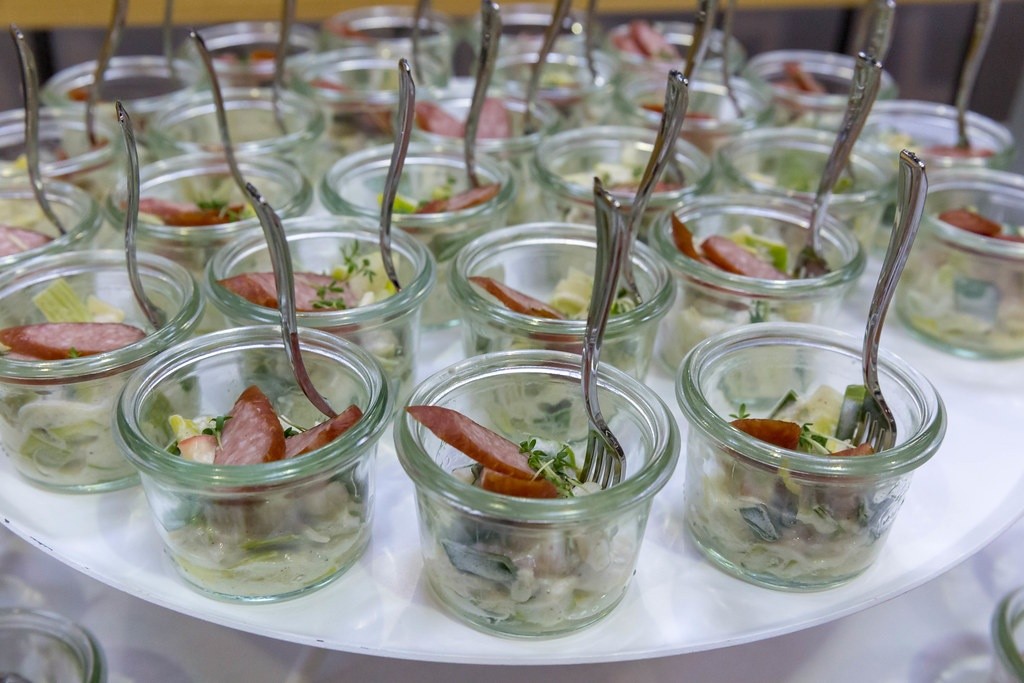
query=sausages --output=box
[0,20,1024,496]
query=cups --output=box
[0,1,1022,642]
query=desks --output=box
[0,74,1024,683]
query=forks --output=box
[792,53,885,279]
[582,174,625,491]
[853,149,929,450]
